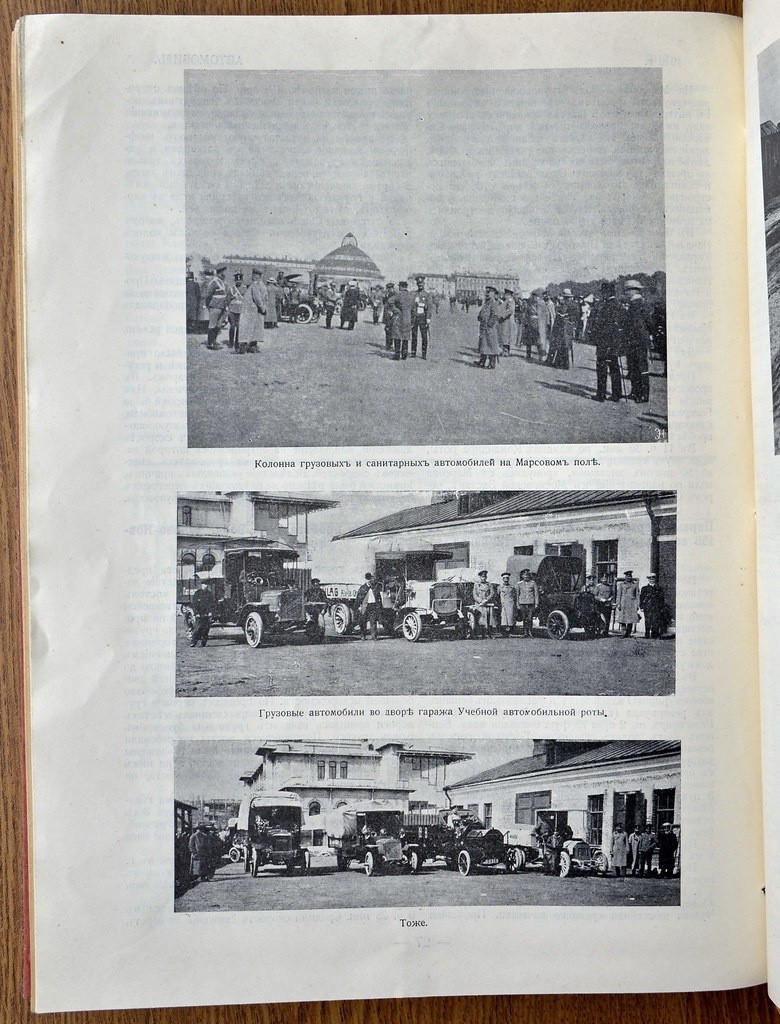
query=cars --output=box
[505,554,607,642]
[184,543,325,649]
[514,807,608,879]
[281,273,320,324]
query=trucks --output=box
[227,817,246,863]
[174,800,198,895]
[236,791,311,879]
[324,799,420,877]
[312,532,478,643]
[403,805,520,877]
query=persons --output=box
[472,569,538,638]
[353,574,384,640]
[473,278,653,403]
[301,578,327,630]
[580,571,666,639]
[610,820,681,878]
[188,823,223,883]
[533,814,571,875]
[204,262,432,362]
[189,582,215,647]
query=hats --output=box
[398,281,408,285]
[415,276,426,282]
[386,283,394,287]
[563,289,574,297]
[502,573,511,578]
[646,573,656,578]
[504,288,514,295]
[252,267,263,274]
[310,578,320,585]
[266,277,275,283]
[478,570,487,576]
[330,281,336,286]
[623,570,633,576]
[520,569,531,574]
[485,286,498,293]
[365,573,373,579]
[623,280,645,290]
[233,273,244,280]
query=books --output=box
[11,0,780,1014]
